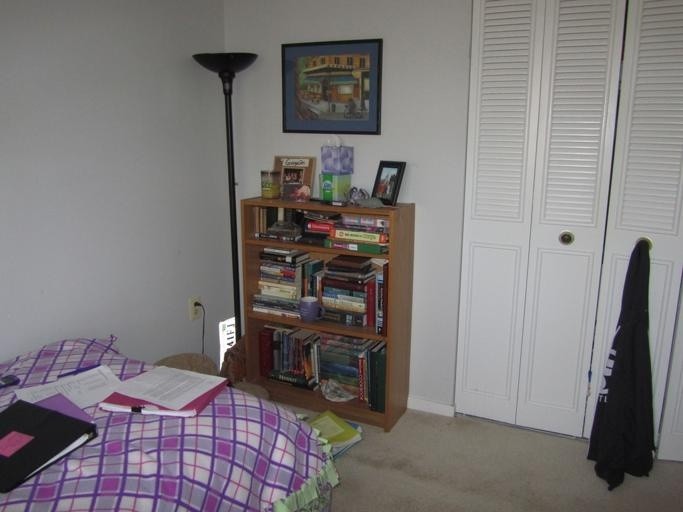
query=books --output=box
[97,377,228,420]
[34,392,96,424]
[251,207,391,462]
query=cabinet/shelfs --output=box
[456,2,683,464]
[240,196,415,434]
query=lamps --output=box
[192,51,269,403]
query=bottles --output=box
[261,171,280,200]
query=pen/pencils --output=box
[57,364,100,378]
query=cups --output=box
[299,297,324,321]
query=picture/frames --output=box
[370,160,408,205]
[281,39,382,135]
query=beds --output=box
[1,335,340,512]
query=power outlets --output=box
[188,295,203,320]
